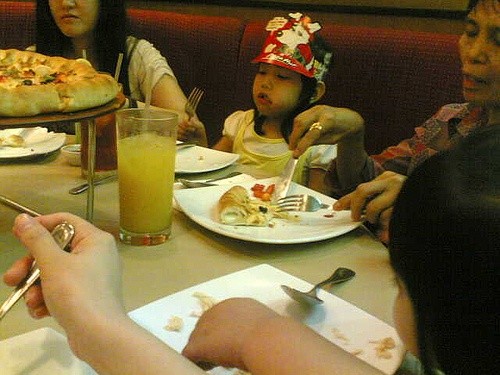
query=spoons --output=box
[281,266,356,305]
[180,171,240,186]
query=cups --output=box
[115,107,178,246]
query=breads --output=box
[0,49,120,116]
[218,185,274,227]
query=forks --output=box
[274,194,385,217]
[184,87,204,121]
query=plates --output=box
[0,327,100,375]
[175,139,240,173]
[0,128,66,159]
[127,263,407,375]
[173,176,368,244]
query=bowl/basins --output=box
[60,144,81,166]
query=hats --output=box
[248,13,333,83]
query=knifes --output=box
[269,128,307,206]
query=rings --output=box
[309,121,325,136]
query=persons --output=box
[176,13,338,198]
[288,0,500,225]
[0,122,500,375]
[25,0,199,126]
